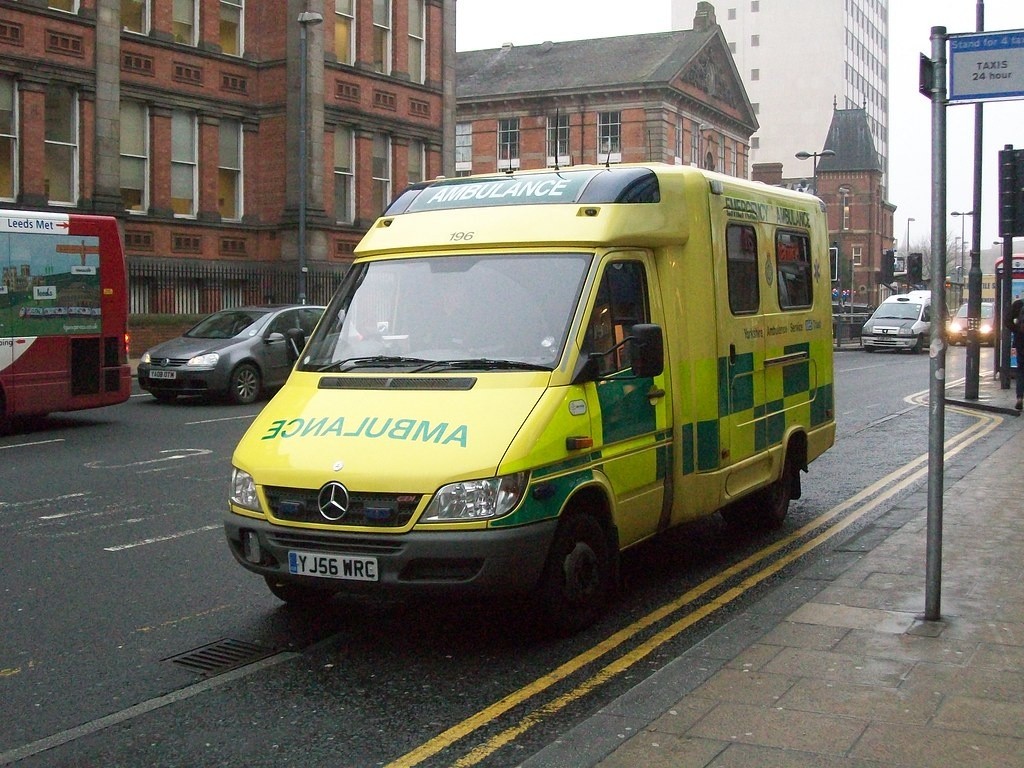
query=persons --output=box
[1005,299,1024,409]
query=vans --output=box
[862,290,952,355]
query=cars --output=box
[138,304,363,405]
[948,302,995,347]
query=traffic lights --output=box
[907,254,922,283]
[829,248,837,281]
[884,251,894,284]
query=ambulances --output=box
[222,162,837,636]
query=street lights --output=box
[994,242,1003,257]
[907,218,915,252]
[955,237,961,269]
[298,12,323,301]
[951,211,973,304]
[795,150,837,195]
[699,124,714,168]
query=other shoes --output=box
[1015,397,1023,409]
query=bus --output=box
[0,210,132,419]
